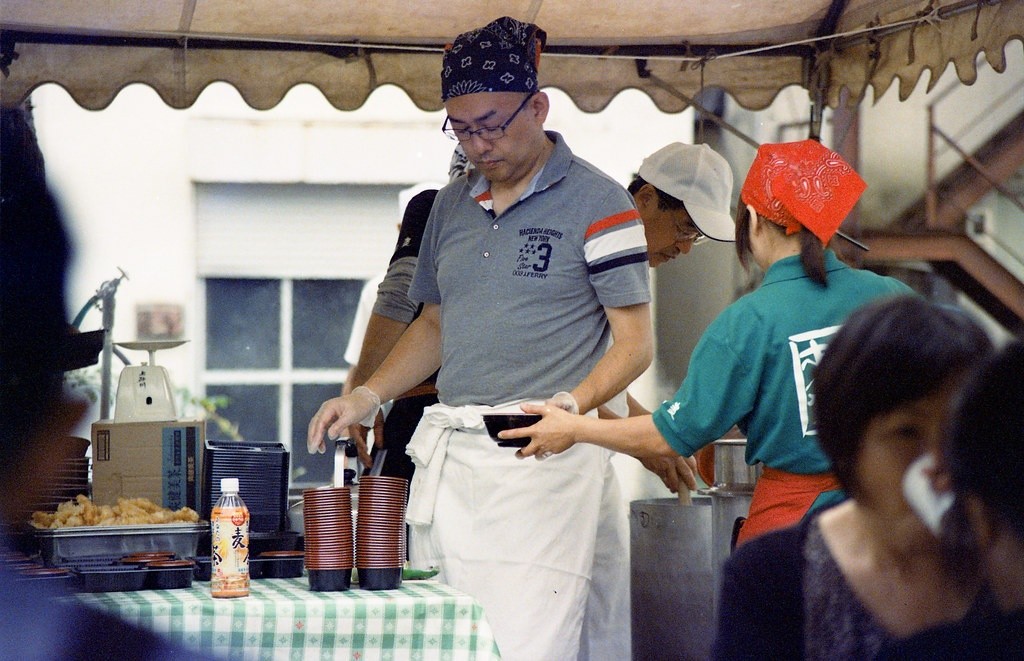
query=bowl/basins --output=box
[0,435,91,558]
[697,444,715,487]
[482,413,543,447]
[301,487,354,591]
[355,476,409,591]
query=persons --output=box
[333,142,476,479]
[307,16,654,661]
[708,296,993,661]
[888,345,1024,661]
[498,139,925,552]
[0,105,211,661]
[578,141,736,661]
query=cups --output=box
[900,453,958,540]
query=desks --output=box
[40,566,504,661]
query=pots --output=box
[714,438,763,491]
[629,497,712,660]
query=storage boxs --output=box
[91,418,207,515]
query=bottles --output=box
[210,478,251,597]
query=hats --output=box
[638,141,737,242]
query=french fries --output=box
[31,494,199,529]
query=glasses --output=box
[441,92,535,141]
[660,198,709,245]
